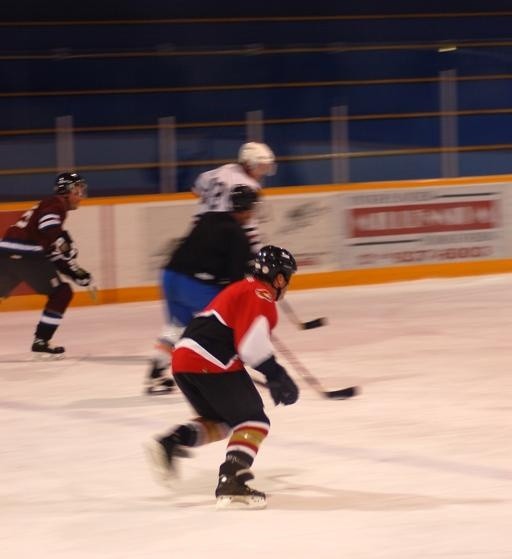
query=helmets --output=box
[226,183,259,211]
[251,246,297,284]
[53,172,89,203]
[238,142,278,176]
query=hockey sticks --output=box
[278,297,329,330]
[271,333,362,400]
[86,285,99,301]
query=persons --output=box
[186,138,276,254]
[142,182,261,388]
[0,171,93,353]
[146,243,301,498]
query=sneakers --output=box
[32,338,64,353]
[159,431,191,465]
[216,468,265,498]
[151,361,175,387]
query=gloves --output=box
[73,267,91,285]
[254,355,297,405]
